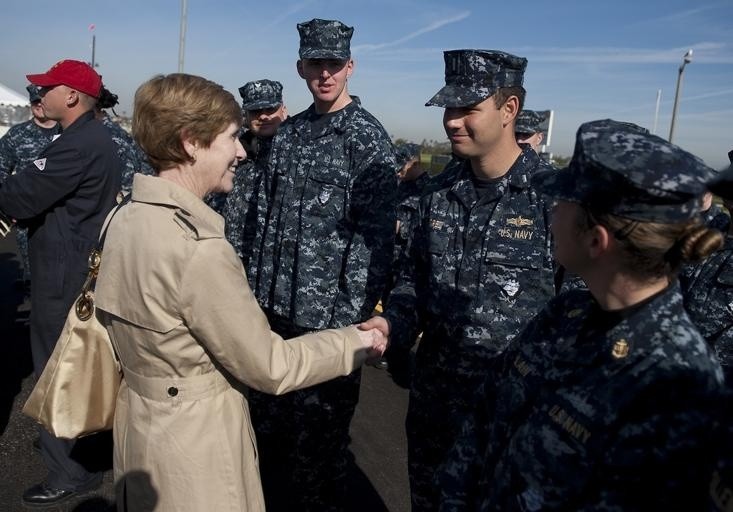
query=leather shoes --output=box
[22,479,77,507]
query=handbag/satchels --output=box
[20,290,122,440]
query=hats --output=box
[296,18,354,60]
[239,79,282,111]
[25,59,103,101]
[530,118,715,222]
[425,50,541,135]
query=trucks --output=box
[428,154,452,176]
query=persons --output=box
[204,79,290,255]
[0,83,63,296]
[358,47,733,512]
[243,18,397,511]
[0,59,123,512]
[93,75,158,193]
[91,73,389,511]
